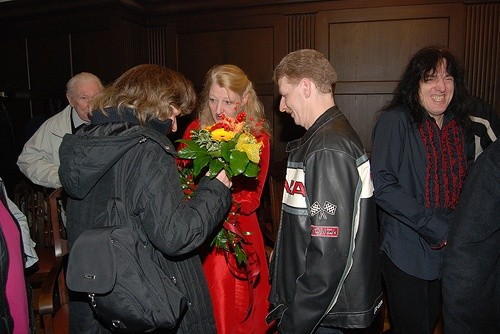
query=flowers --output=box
[175,122,264,264]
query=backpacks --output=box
[66,144,190,334]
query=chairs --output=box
[13,181,68,334]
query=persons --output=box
[175,65,272,334]
[368,46,500,334]
[265,49,385,334]
[16,71,106,228]
[59,65,233,334]
[441,138,500,334]
